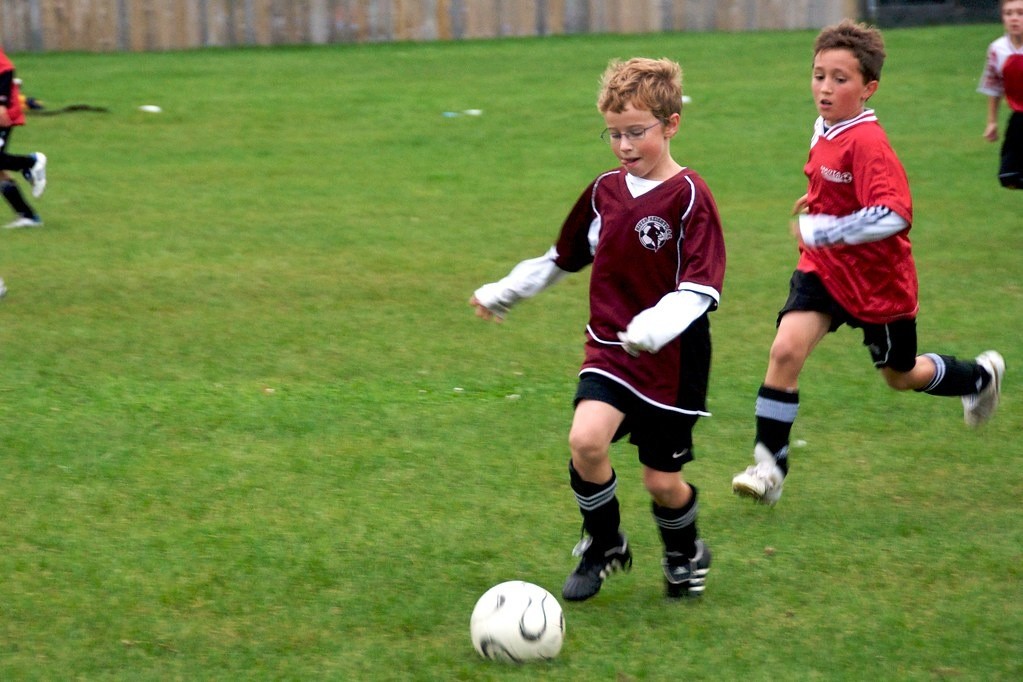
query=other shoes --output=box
[23,152,47,198]
[5,213,43,229]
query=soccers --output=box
[469,580,567,669]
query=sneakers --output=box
[962,350,1005,434]
[662,537,711,601]
[562,531,632,602]
[731,442,785,511]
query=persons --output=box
[0,45,47,231]
[731,19,1006,510]
[977,0,1023,191]
[468,56,726,602]
[0,275,7,297]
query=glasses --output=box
[600,119,669,144]
